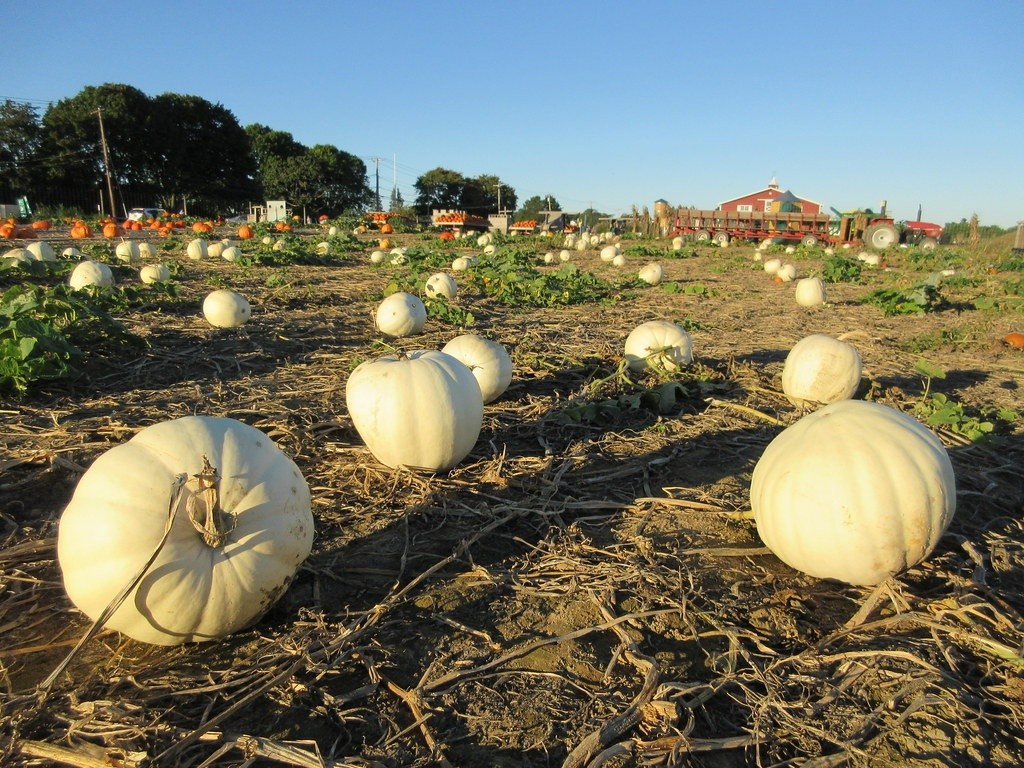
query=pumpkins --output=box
[1,210,1023,641]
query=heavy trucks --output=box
[667,205,944,253]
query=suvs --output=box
[129,207,169,222]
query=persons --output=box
[217,214,222,223]
[179,209,184,214]
